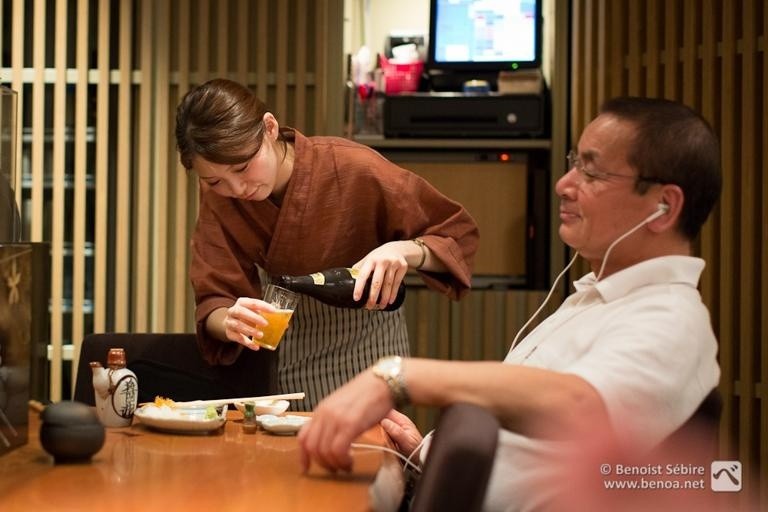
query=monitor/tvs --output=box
[429,0,541,69]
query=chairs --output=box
[672,388,721,468]
[75,333,278,410]
[402,403,498,512]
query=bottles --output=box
[278,267,407,311]
[391,44,419,65]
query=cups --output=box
[252,284,301,352]
[37,399,106,467]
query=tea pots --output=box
[90,348,138,428]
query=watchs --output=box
[371,354,413,409]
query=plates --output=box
[134,392,311,436]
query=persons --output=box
[171,76,481,416]
[298,93,723,512]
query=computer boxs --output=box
[381,90,544,139]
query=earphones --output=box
[656,202,671,215]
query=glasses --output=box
[565,149,665,186]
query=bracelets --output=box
[411,239,427,271]
